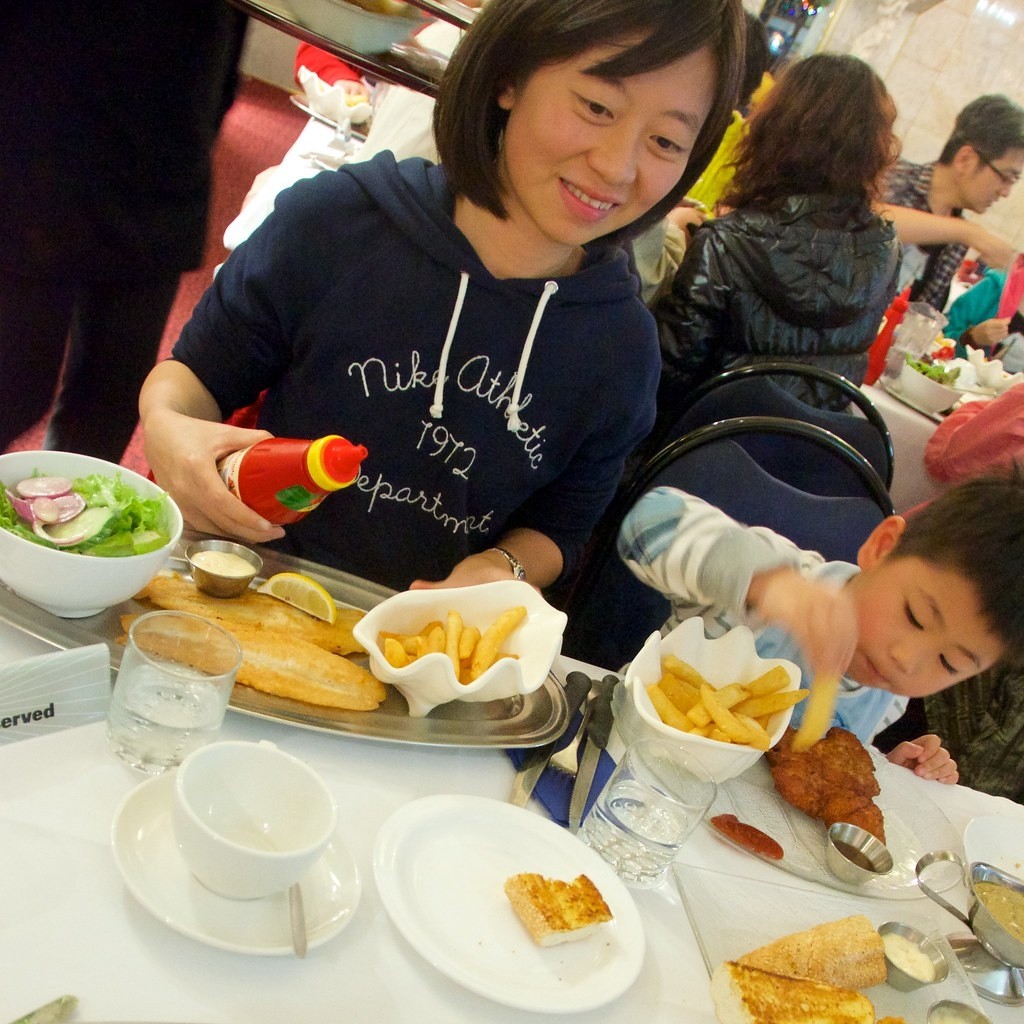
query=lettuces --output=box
[0,473,169,558]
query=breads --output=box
[504,871,613,947]
[707,913,888,1024]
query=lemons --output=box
[257,571,338,626]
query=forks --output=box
[547,679,601,781]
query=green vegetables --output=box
[903,354,961,387]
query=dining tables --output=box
[860,304,1024,516]
[0,493,1024,1024]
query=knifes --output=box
[507,671,593,809]
[569,675,621,836]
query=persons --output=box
[0,1,251,466]
[612,465,1024,781]
[136,1,746,604]
[632,18,1024,488]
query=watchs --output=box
[487,548,526,582]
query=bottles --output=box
[150,435,368,528]
[863,287,912,385]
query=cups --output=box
[916,852,1024,970]
[171,739,337,902]
[878,298,950,386]
[107,611,246,774]
[583,738,720,890]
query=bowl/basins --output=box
[877,921,949,993]
[827,822,895,886]
[926,999,993,1024]
[185,540,264,599]
[896,360,964,414]
[288,0,438,56]
[0,451,184,621]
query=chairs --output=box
[557,413,897,674]
[649,360,894,492]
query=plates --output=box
[1,537,574,748]
[289,95,368,141]
[391,44,449,81]
[964,812,1024,881]
[671,861,990,1024]
[966,343,1024,394]
[371,795,646,1013]
[442,0,480,20]
[110,775,363,957]
[611,661,966,901]
[879,376,949,424]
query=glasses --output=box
[972,148,1021,185]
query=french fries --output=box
[791,676,841,753]
[374,603,528,688]
[648,653,809,753]
